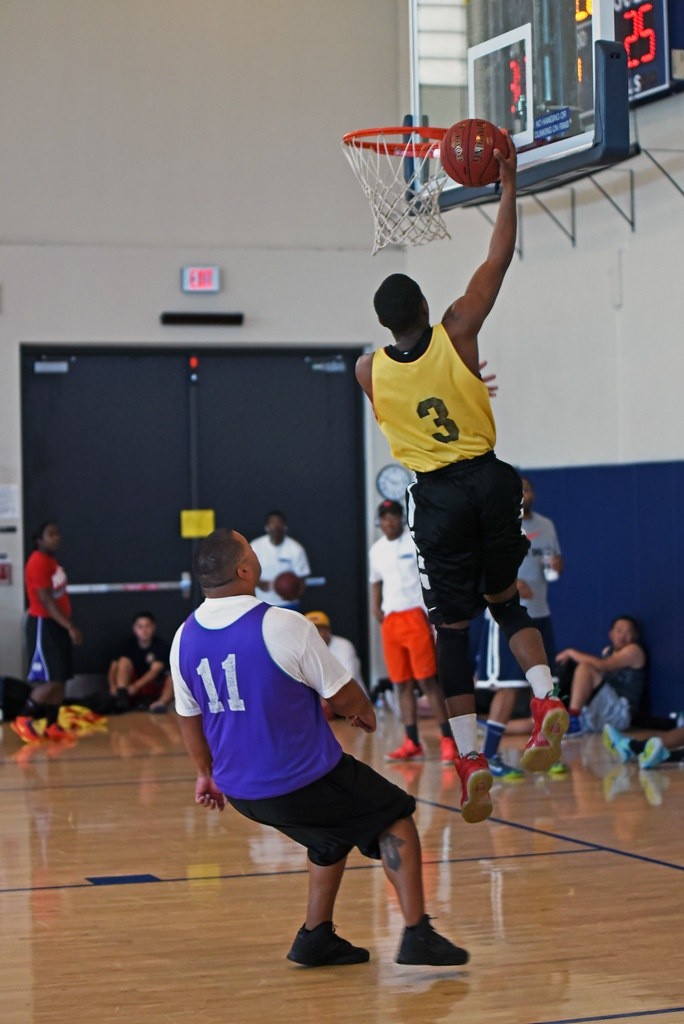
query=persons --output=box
[356,131,570,824]
[475,477,569,780]
[556,615,651,739]
[304,611,366,720]
[10,521,83,744]
[169,527,470,966]
[106,612,174,713]
[249,510,310,611]
[602,722,684,768]
[369,500,459,764]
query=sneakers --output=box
[561,716,593,738]
[601,722,636,765]
[396,914,468,965]
[454,750,493,823]
[383,738,425,762]
[9,714,42,744]
[286,920,370,966]
[44,723,76,743]
[486,752,528,784]
[637,736,671,769]
[437,736,458,765]
[519,683,570,773]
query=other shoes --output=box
[114,692,131,712]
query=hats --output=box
[377,500,403,516]
[302,610,331,627]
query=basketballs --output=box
[273,571,304,600]
[440,119,510,188]
